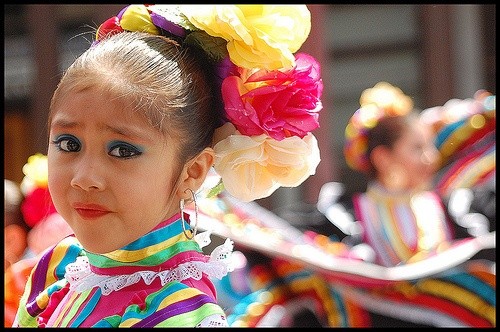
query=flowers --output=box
[183,5,323,202]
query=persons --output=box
[10,32,230,329]
[3,178,37,327]
[345,80,453,274]
